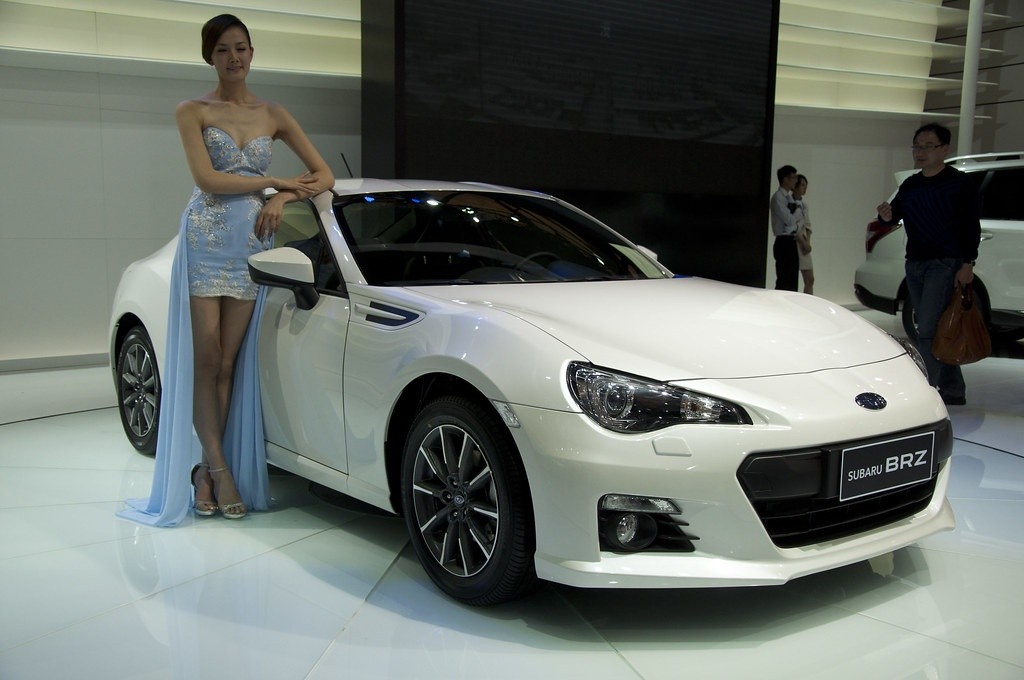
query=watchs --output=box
[963,260,976,266]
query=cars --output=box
[103,174,953,611]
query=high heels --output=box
[208,466,246,519]
[190,462,217,516]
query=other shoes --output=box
[938,390,966,405]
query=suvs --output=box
[853,150,1024,354]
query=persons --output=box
[877,122,982,404]
[176,14,335,519]
[790,173,814,295]
[771,166,812,291]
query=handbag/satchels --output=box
[931,280,991,364]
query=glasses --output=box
[911,143,945,152]
